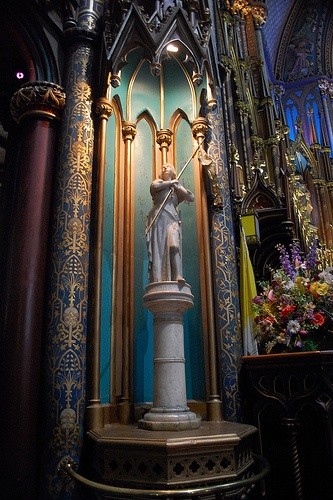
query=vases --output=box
[238,350,333,427]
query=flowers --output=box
[250,236,333,354]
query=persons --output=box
[147,163,196,285]
